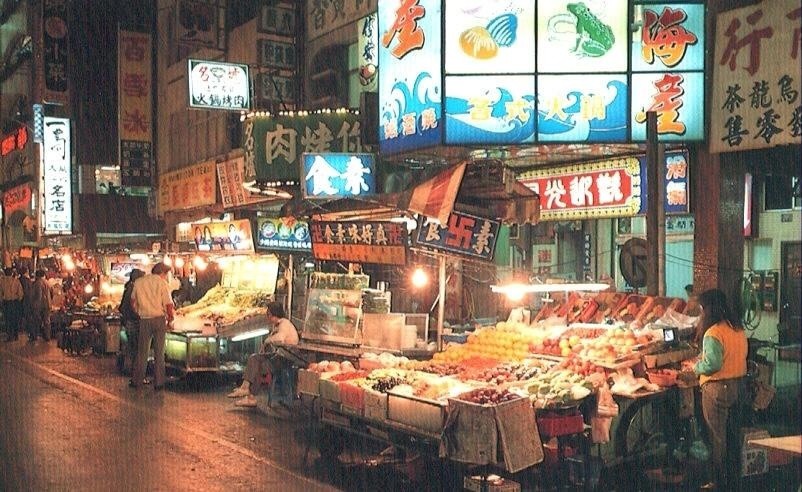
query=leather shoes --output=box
[700,482,723,491]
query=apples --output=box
[581,328,652,362]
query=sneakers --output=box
[236,397,258,407]
[129,377,163,390]
[227,388,250,397]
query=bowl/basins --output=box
[646,369,678,386]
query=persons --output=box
[97,180,128,197]
[0,264,95,346]
[685,285,693,296]
[680,288,748,492]
[226,302,299,407]
[118,262,197,392]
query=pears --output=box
[526,370,593,410]
[558,335,579,357]
